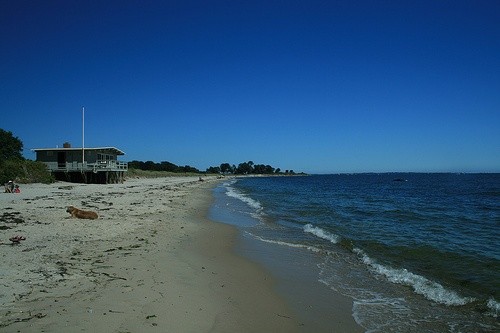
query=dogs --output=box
[65,206,99,220]
